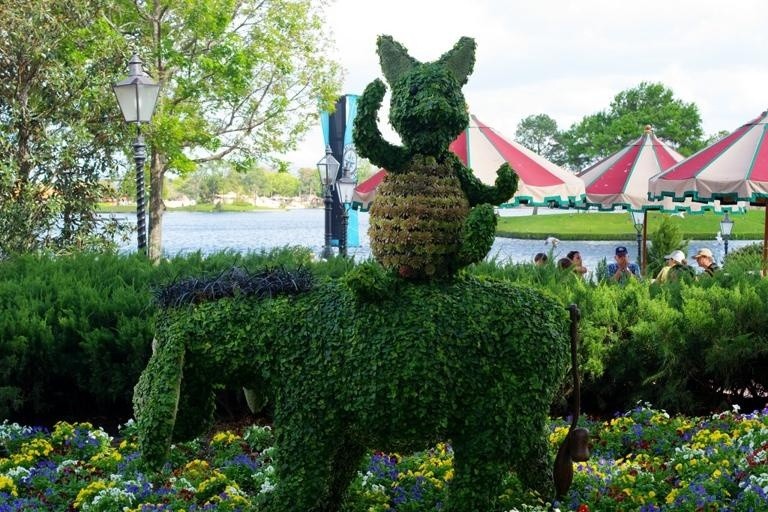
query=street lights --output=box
[315,143,340,260]
[334,166,358,255]
[720,208,735,256]
[630,211,646,266]
[114,51,161,256]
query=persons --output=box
[653,249,698,283]
[534,253,547,267]
[604,246,641,286]
[556,258,571,273]
[691,248,719,277]
[567,250,587,274]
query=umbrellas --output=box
[547,123,732,275]
[646,110,768,275]
[348,108,584,211]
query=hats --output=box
[615,247,628,256]
[691,248,713,259]
[664,249,685,264]
